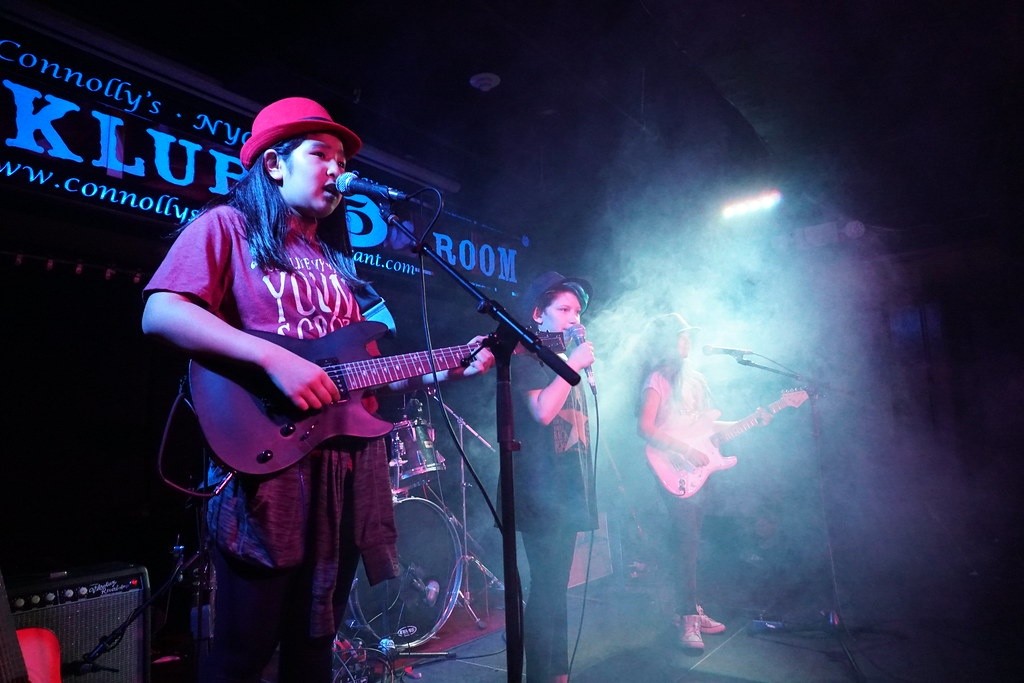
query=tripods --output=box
[737,357,909,683]
[362,389,525,682]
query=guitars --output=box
[188,320,566,476]
[644,385,811,498]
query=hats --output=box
[522,272,593,325]
[240,96,363,171]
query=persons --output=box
[636,313,772,651]
[494,271,599,683]
[142,96,496,683]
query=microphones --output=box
[336,172,412,201]
[569,324,597,395]
[702,345,752,357]
[422,578,441,607]
[58,660,102,680]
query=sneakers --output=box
[680,615,704,649]
[675,604,725,633]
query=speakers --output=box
[6,561,152,683]
[568,505,613,590]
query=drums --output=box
[387,418,446,495]
[341,492,465,650]
